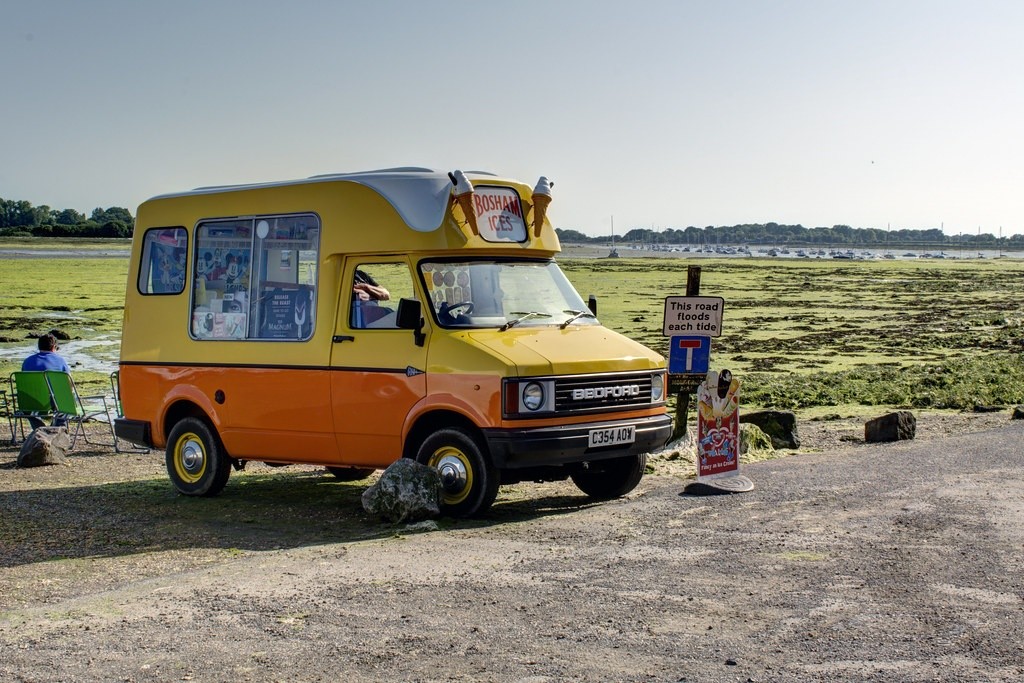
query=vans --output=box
[113,164,677,524]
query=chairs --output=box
[0,371,151,454]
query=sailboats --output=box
[632,225,752,255]
[902,223,1008,260]
[758,223,896,260]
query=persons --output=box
[22,334,72,431]
[352,268,389,305]
